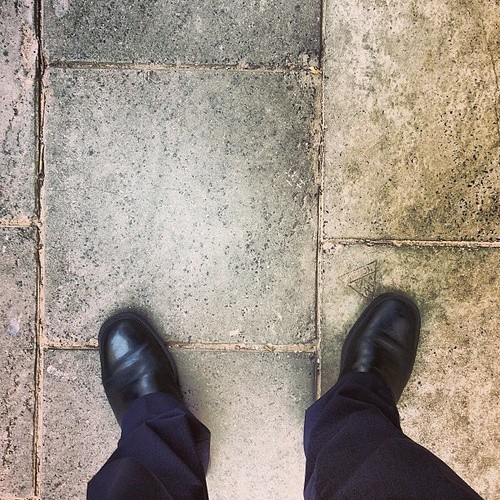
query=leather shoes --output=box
[339,292,422,405]
[98,312,182,437]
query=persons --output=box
[86,292,484,500]
